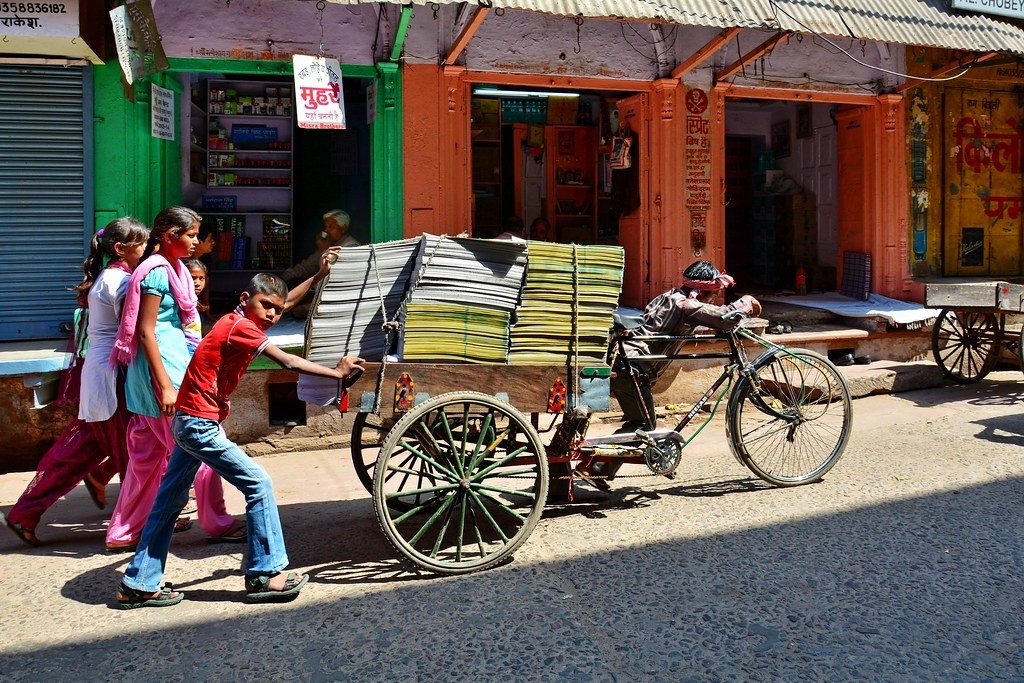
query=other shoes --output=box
[574,463,612,493]
[80,472,111,511]
[779,319,794,333]
[174,516,193,533]
[854,355,871,365]
[180,501,197,514]
[3,513,41,548]
[768,321,784,335]
[835,353,856,366]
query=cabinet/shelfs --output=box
[191,102,207,208]
[552,125,599,243]
[472,97,504,238]
[205,78,295,302]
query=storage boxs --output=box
[202,194,237,213]
[209,87,291,187]
[774,240,817,257]
[805,267,835,292]
[231,236,251,270]
[724,133,783,288]
[211,216,228,234]
[776,210,817,227]
[256,215,289,270]
[775,225,818,241]
[228,215,246,237]
[776,256,817,269]
[774,194,816,212]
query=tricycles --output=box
[922,281,1024,386]
[330,307,855,577]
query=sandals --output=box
[115,581,184,611]
[244,571,309,603]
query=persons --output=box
[573,260,762,492]
[0,206,249,552]
[277,209,362,317]
[114,245,341,608]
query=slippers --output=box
[203,520,247,543]
[106,537,140,552]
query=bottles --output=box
[542,102,547,123]
[512,101,518,122]
[537,102,542,124]
[586,101,592,126]
[531,102,537,123]
[1002,289,1010,309]
[506,101,512,122]
[578,102,586,125]
[518,101,524,122]
[525,101,531,122]
[796,260,806,290]
[501,101,505,123]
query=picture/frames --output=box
[796,103,813,139]
[771,119,791,160]
